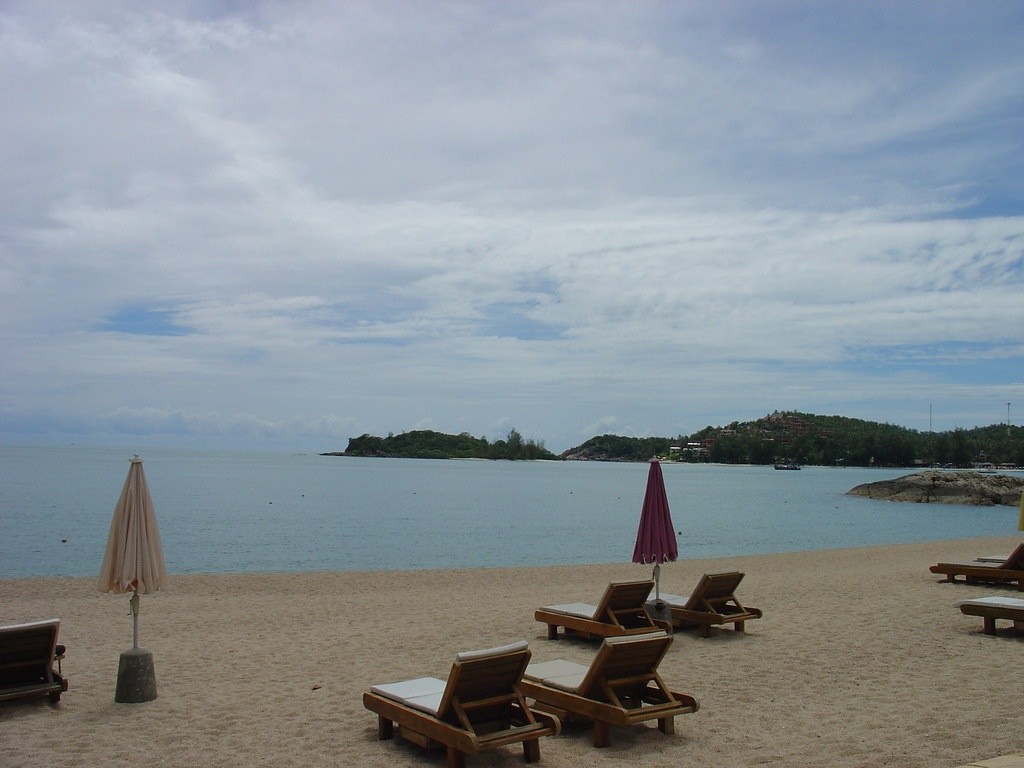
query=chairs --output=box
[929,541,1023,592]
[972,554,1009,563]
[951,595,1023,635]
[519,628,702,749]
[362,638,561,767]
[535,577,673,646]
[644,569,763,640]
[0,617,69,705]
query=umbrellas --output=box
[630,454,679,599]
[95,452,168,650]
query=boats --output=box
[774,462,801,471]
